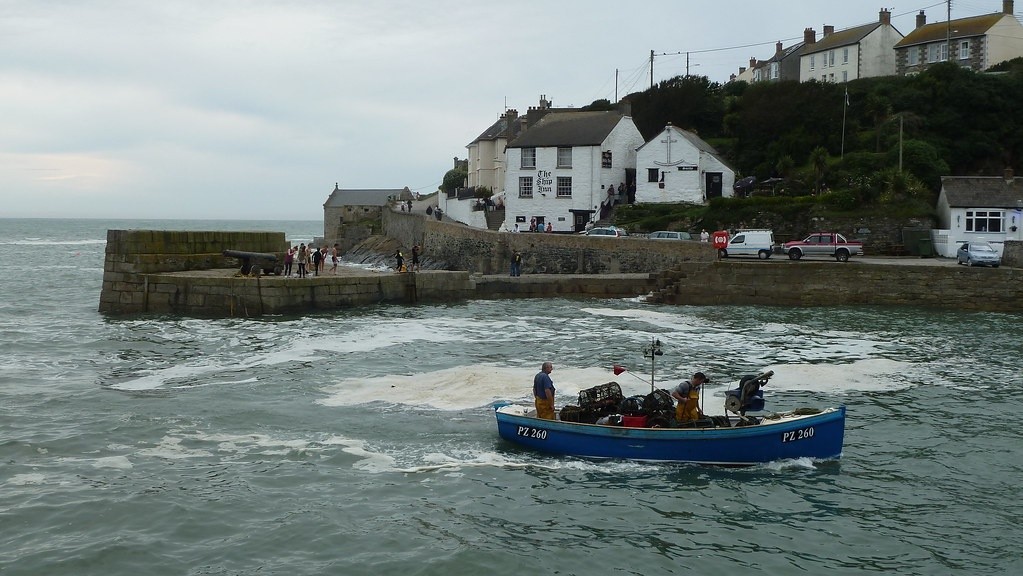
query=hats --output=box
[693,372,706,379]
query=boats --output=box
[493,402,847,464]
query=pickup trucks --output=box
[780,232,864,262]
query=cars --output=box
[956,241,1000,268]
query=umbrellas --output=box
[733,176,756,197]
[759,177,784,196]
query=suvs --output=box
[578,226,630,238]
[646,230,693,240]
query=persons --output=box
[533,362,557,419]
[283,242,342,278]
[395,250,404,272]
[585,180,635,231]
[778,176,854,197]
[672,373,706,422]
[481,196,552,233]
[412,245,420,272]
[511,249,521,276]
[700,224,736,258]
[401,200,443,221]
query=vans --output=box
[718,228,776,260]
